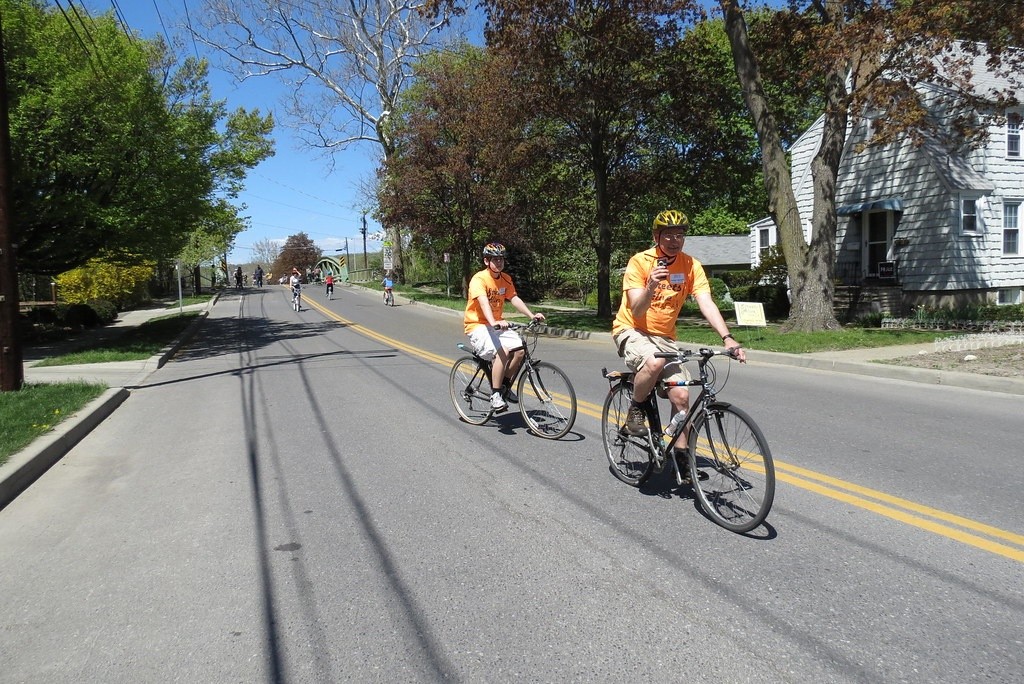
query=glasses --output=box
[660,234,684,241]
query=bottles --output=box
[665,409,686,437]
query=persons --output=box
[464,243,546,409]
[380,273,393,305]
[613,210,746,480]
[210,264,335,307]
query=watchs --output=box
[722,334,734,342]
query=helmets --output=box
[328,272,332,275]
[385,273,391,278]
[652,210,689,230]
[483,243,505,257]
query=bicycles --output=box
[290,286,306,311]
[238,282,242,290]
[327,287,332,300]
[381,285,394,307]
[448,317,577,440]
[602,342,775,533]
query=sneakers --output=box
[490,392,509,413]
[672,447,709,480]
[505,390,518,404]
[625,404,649,437]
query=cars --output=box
[278,275,290,285]
